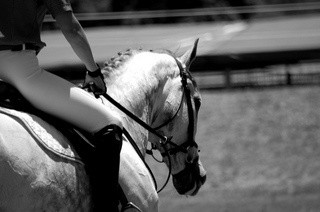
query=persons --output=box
[0,0,134,212]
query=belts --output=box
[0,43,39,55]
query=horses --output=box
[0,37,208,212]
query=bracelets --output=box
[84,64,101,76]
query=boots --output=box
[90,124,122,212]
